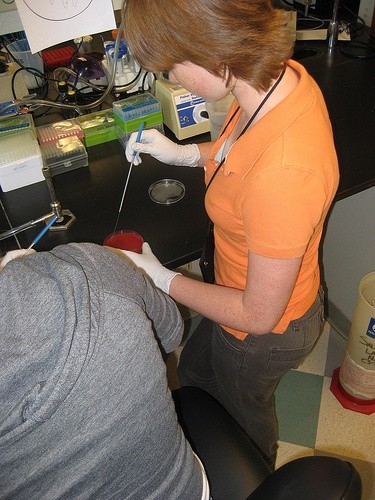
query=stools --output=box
[324,178,375,337]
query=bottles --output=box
[103,29,135,75]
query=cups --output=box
[1,38,44,89]
[76,39,92,54]
[346,271,375,371]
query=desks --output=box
[0,0,375,290]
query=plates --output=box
[148,179,185,204]
[103,230,144,255]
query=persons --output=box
[0,243,212,500]
[103,0,341,468]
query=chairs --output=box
[171,385,362,500]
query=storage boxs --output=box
[0,79,211,193]
